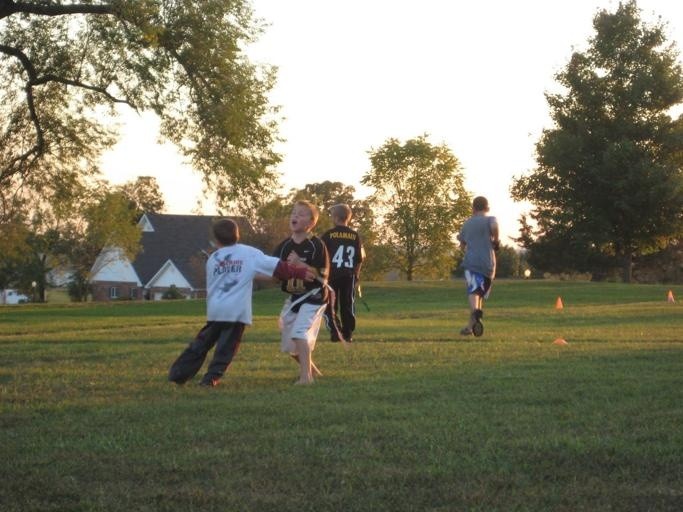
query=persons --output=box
[321,204,365,343]
[168,218,315,387]
[459,196,500,337]
[272,198,330,386]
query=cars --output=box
[1,287,30,305]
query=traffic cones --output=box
[553,294,563,309]
[667,288,674,304]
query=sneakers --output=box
[473,310,484,336]
[461,327,473,335]
